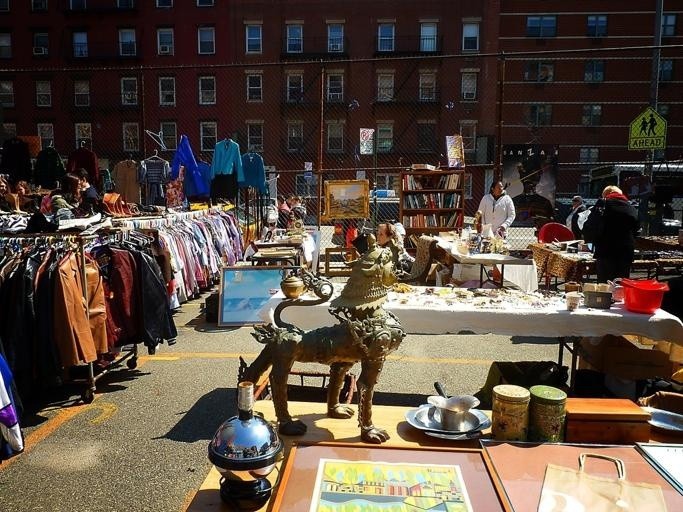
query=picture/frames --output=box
[322,178,372,221]
[268,439,513,511]
[217,264,297,329]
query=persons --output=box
[0,167,99,217]
[582,185,641,285]
[565,196,587,240]
[475,181,516,239]
[276,193,307,231]
[449,159,461,167]
[376,222,405,266]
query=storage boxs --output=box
[565,398,652,448]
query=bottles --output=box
[492,384,531,441]
[529,385,567,442]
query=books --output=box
[402,174,462,249]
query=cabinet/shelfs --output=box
[398,169,467,256]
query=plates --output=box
[404,404,492,434]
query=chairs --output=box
[400,235,437,285]
[537,223,576,286]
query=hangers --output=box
[0,129,260,265]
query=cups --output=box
[566,295,581,312]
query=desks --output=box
[265,279,680,392]
[186,398,683,511]
[528,241,683,290]
[637,230,682,250]
[244,224,322,275]
[424,234,535,288]
